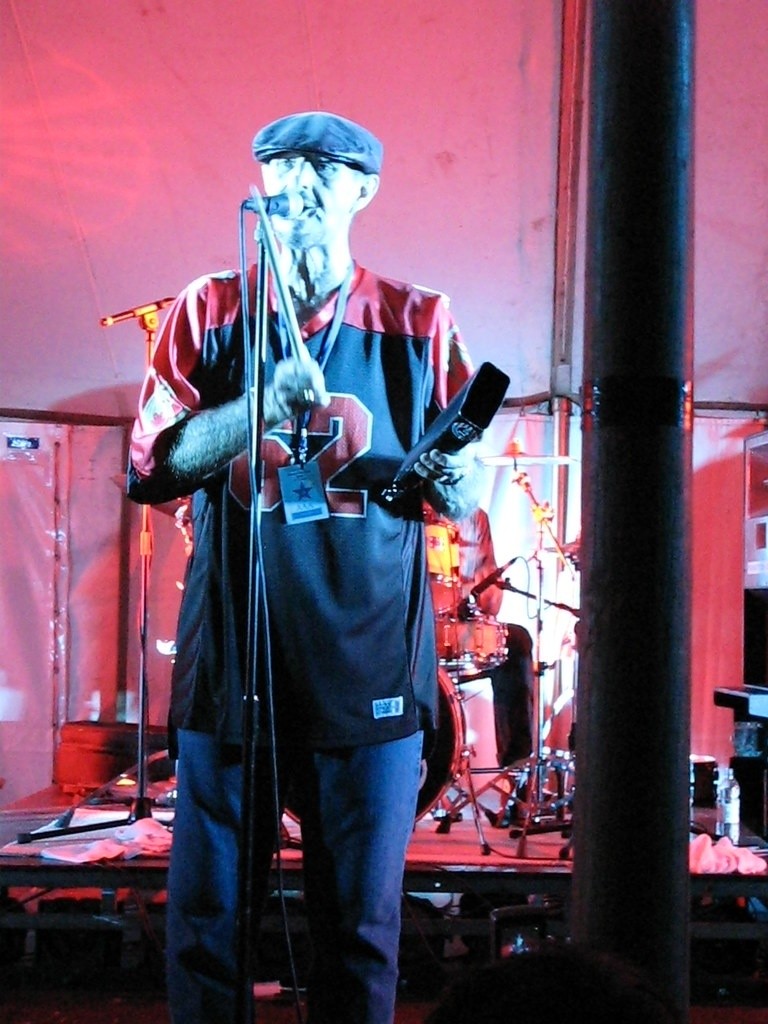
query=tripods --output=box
[17,295,181,842]
[435,461,580,858]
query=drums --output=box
[284,661,464,837]
[422,516,461,613]
[435,612,509,669]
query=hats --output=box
[251,111,382,173]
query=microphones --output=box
[243,191,304,218]
[470,557,518,598]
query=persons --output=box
[127,111,489,1024]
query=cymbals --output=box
[476,456,576,465]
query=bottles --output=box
[716,769,740,845]
